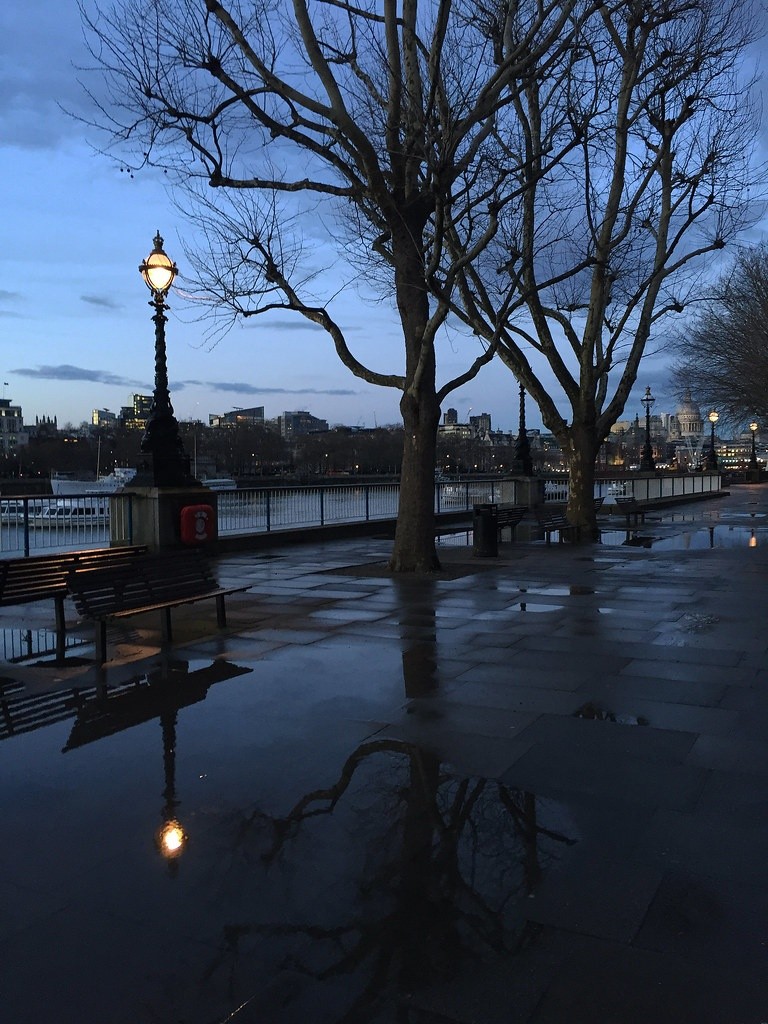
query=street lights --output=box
[512,372,537,477]
[638,385,659,473]
[701,407,719,466]
[747,420,760,469]
[123,230,206,487]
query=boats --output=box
[439,485,498,505]
[1,467,139,528]
[201,480,238,491]
[545,484,568,499]
[608,483,628,496]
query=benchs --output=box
[62,548,253,664]
[615,497,656,528]
[531,508,582,546]
[493,505,529,543]
[0,544,149,633]
[566,497,606,514]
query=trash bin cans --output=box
[472,503,498,556]
